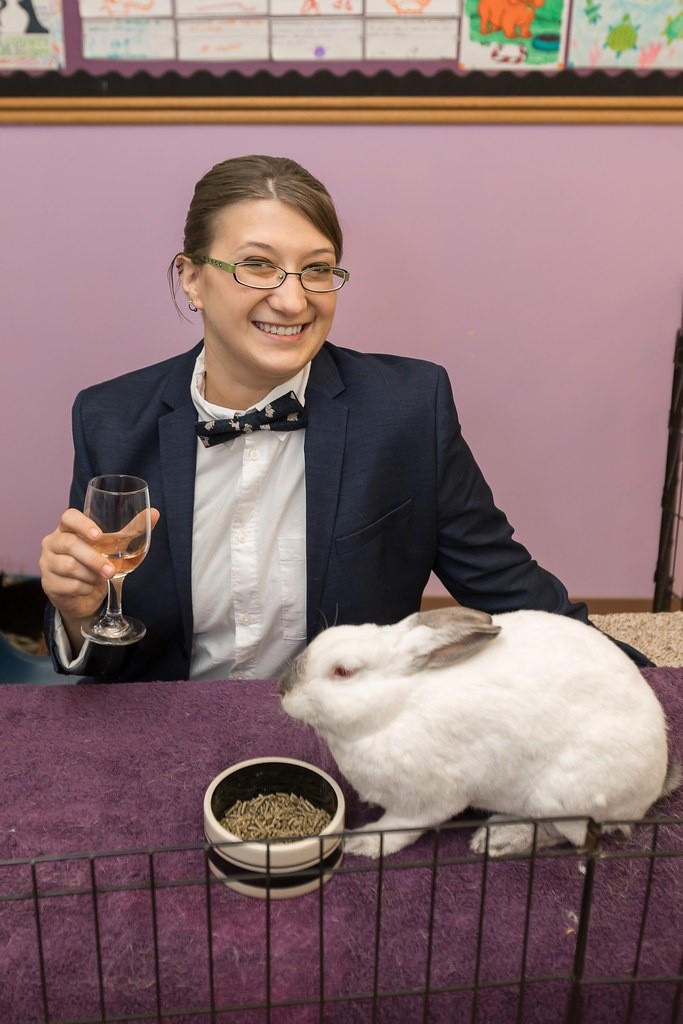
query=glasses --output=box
[198,254,350,293]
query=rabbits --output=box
[272,604,683,861]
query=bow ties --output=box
[194,390,306,448]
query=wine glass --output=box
[81,474,151,647]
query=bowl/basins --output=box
[202,757,345,900]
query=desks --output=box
[0,664,683,1024]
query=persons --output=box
[38,153,659,685]
